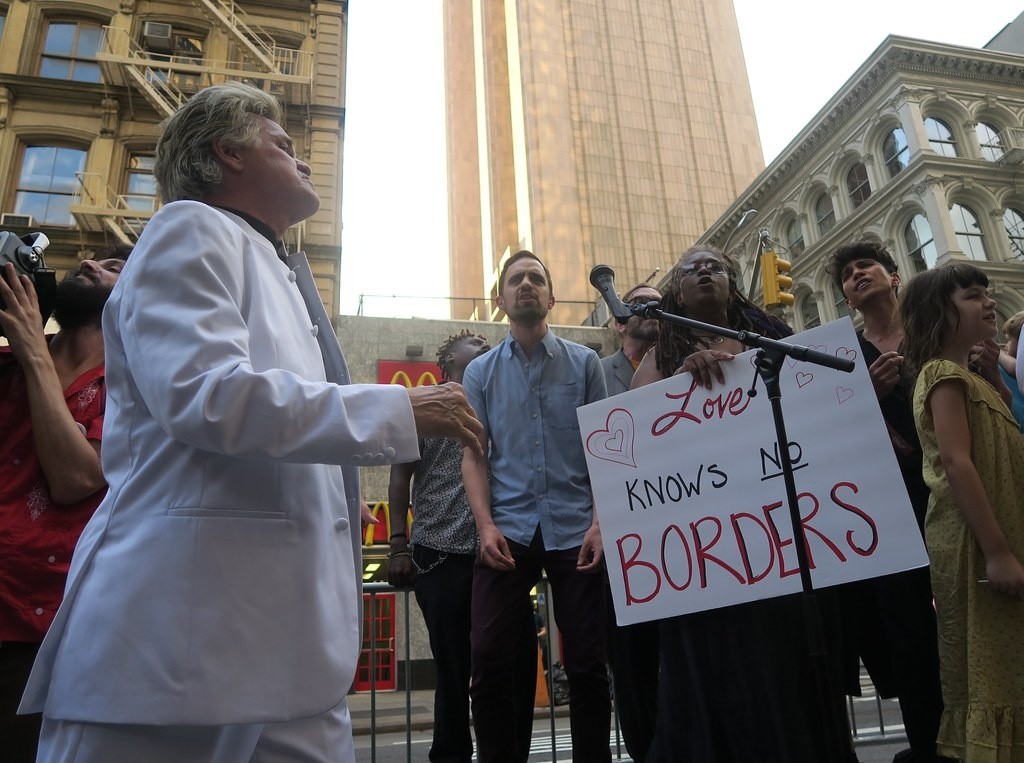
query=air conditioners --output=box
[1,213,40,227]
[144,22,175,50]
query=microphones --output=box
[590,264,629,325]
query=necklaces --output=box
[697,335,724,344]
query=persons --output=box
[970,311,1024,426]
[600,284,662,763]
[461,250,611,763]
[632,247,857,763]
[0,246,136,763]
[387,329,492,763]
[897,263,1024,763]
[17,82,485,763]
[833,241,946,763]
[533,610,547,670]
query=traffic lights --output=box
[760,250,794,309]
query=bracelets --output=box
[389,533,406,540]
[387,551,409,558]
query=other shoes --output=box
[893,748,912,763]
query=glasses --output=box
[628,295,663,304]
[677,261,728,275]
